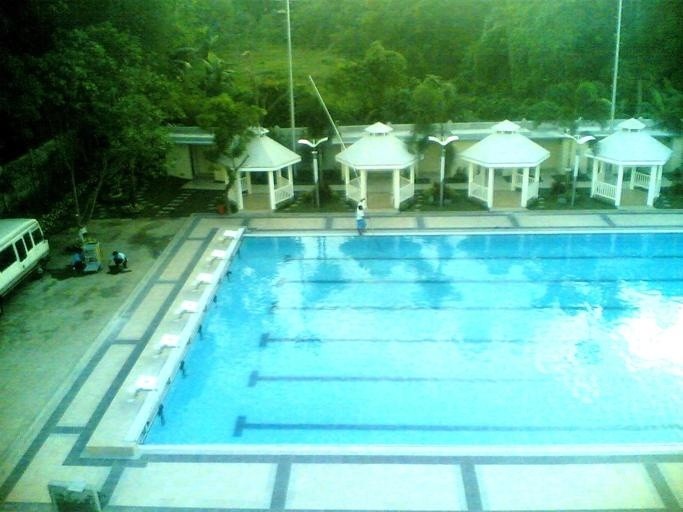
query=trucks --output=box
[0,214,50,314]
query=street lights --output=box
[298,134,329,208]
[426,134,458,208]
[564,132,596,207]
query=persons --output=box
[356,198,367,235]
[112,251,127,268]
[72,248,83,270]
